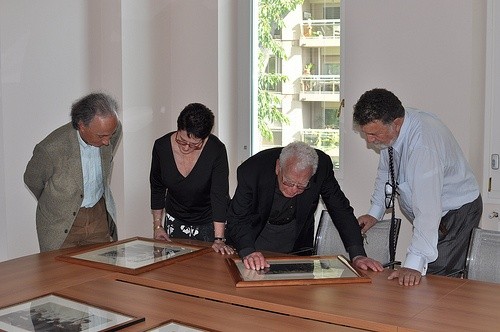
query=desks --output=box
[0,238,500,332]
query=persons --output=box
[352,87,483,287]
[224,139,386,272]
[150,101,235,255]
[23,93,124,255]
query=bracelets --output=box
[152,216,162,225]
[214,237,227,243]
[154,225,162,230]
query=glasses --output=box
[385,182,401,209]
[281,168,311,190]
[175,131,203,149]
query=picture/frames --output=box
[143,319,223,332]
[224,255,372,288]
[54,236,215,276]
[0,292,145,332]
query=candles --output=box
[303,62,314,75]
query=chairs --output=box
[444,227,500,284]
[313,210,402,269]
